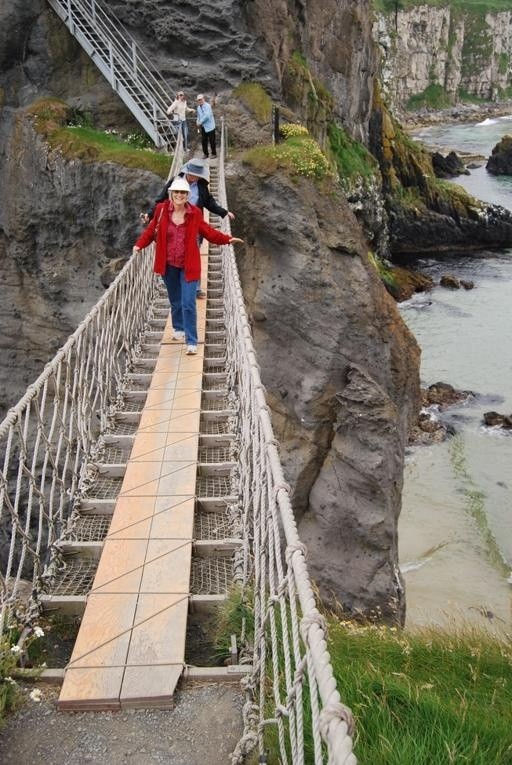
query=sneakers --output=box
[202,154,208,159]
[171,330,186,340]
[210,154,216,159]
[186,345,197,355]
[197,290,207,299]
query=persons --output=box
[195,94,217,159]
[140,156,236,297]
[167,91,195,152]
[132,178,245,355]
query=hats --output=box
[180,158,209,179]
[168,178,190,192]
[197,94,204,100]
